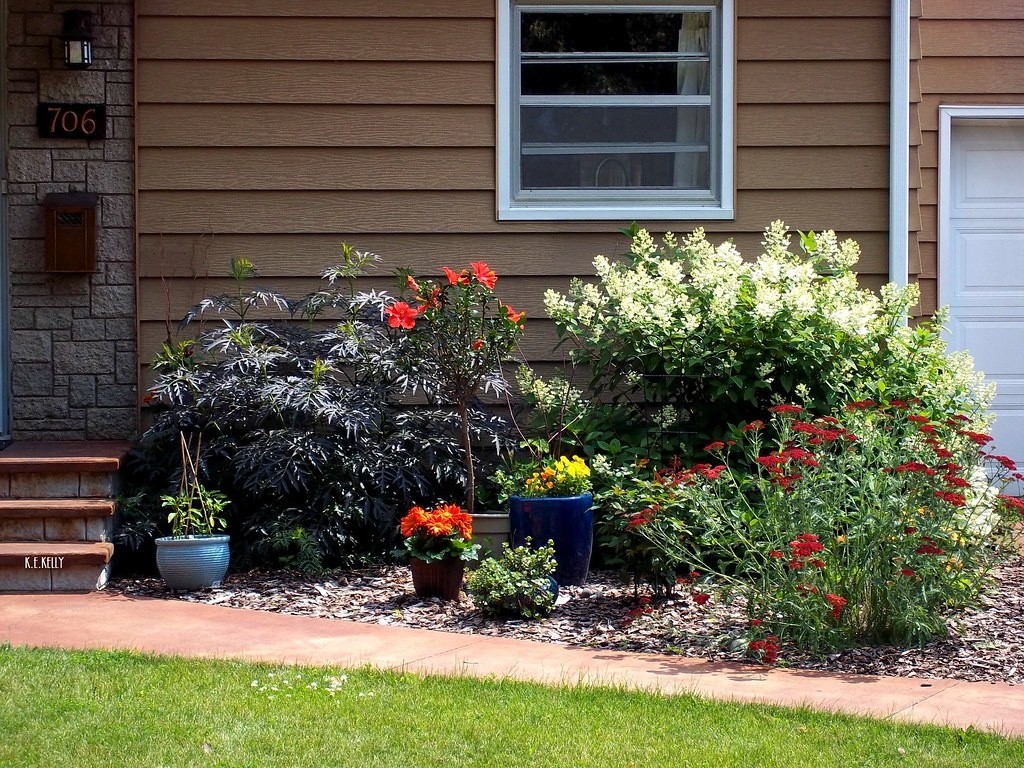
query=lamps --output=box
[57,9,97,69]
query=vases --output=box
[510,492,593,586]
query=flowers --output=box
[523,455,593,499]
[401,503,482,562]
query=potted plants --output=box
[464,535,559,619]
[155,431,230,589]
[409,557,465,600]
